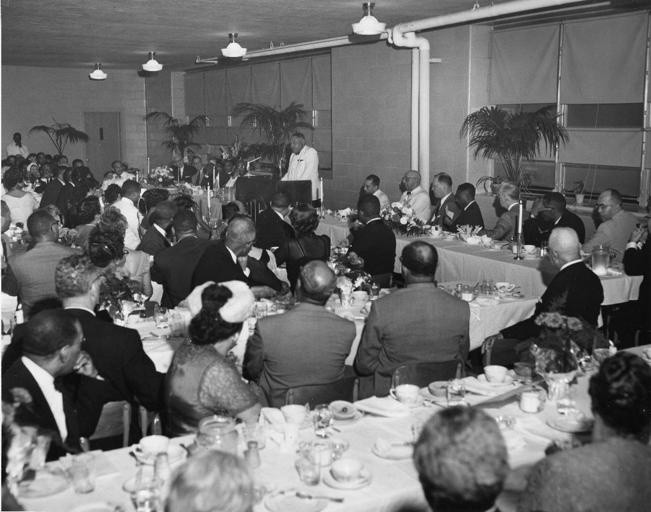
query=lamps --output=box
[142,50,164,71]
[351,2,388,36]
[88,63,108,80]
[220,33,249,58]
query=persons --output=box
[397,170,650,353]
[164,352,651,512]
[2,226,470,502]
[1,133,396,334]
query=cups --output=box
[453,278,522,305]
[15,365,592,511]
[246,286,393,330]
[424,225,611,275]
